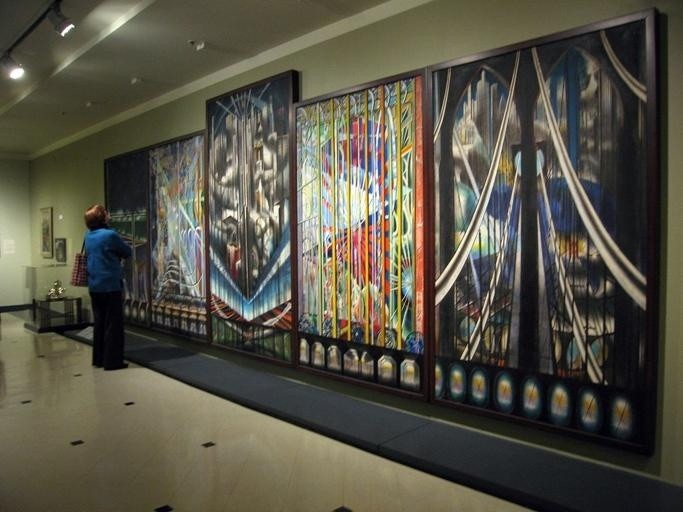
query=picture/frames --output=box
[39,206,66,262]
[426,12,663,454]
[150,130,208,347]
[297,65,426,399]
[103,144,150,336]
[203,71,295,368]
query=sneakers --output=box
[94,359,129,371]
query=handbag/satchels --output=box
[72,236,88,287]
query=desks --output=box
[33,296,81,326]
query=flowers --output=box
[48,280,65,297]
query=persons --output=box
[84,204,132,370]
